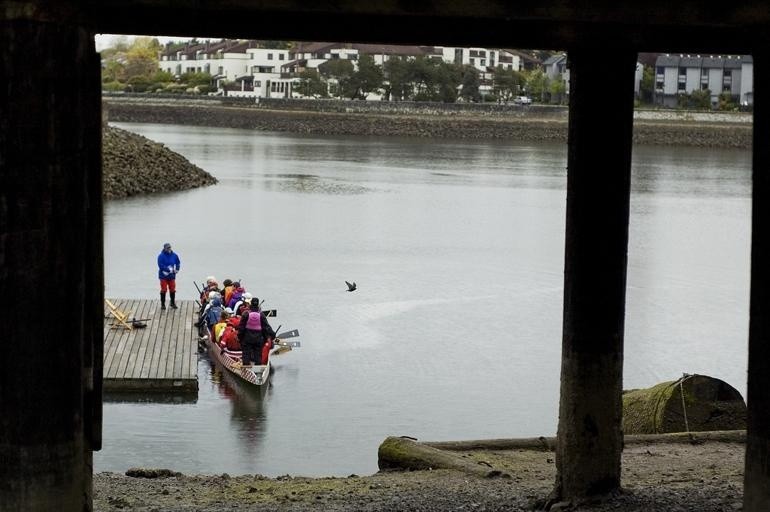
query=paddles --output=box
[262,310,277,317]
[274,341,300,347]
[275,329,299,339]
[271,346,292,355]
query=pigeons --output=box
[345,281,357,293]
[132,318,146,330]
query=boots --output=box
[160,292,166,310]
[169,291,178,309]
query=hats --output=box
[163,243,172,250]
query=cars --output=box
[513,96,532,104]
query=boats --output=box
[197,274,281,387]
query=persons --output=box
[237,298,274,365]
[200,277,273,363]
[158,243,180,310]
